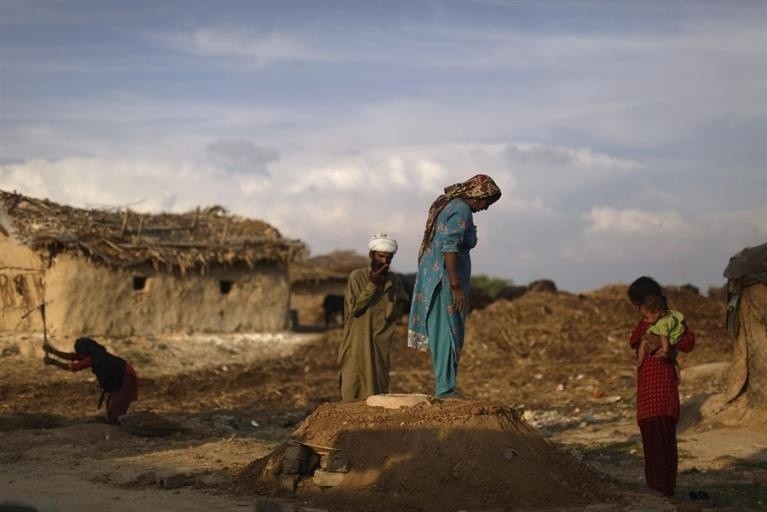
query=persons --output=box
[629,274,695,496]
[41,337,140,425]
[636,295,686,371]
[338,232,411,401]
[408,173,503,398]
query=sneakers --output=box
[434,387,486,404]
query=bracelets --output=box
[451,282,464,291]
